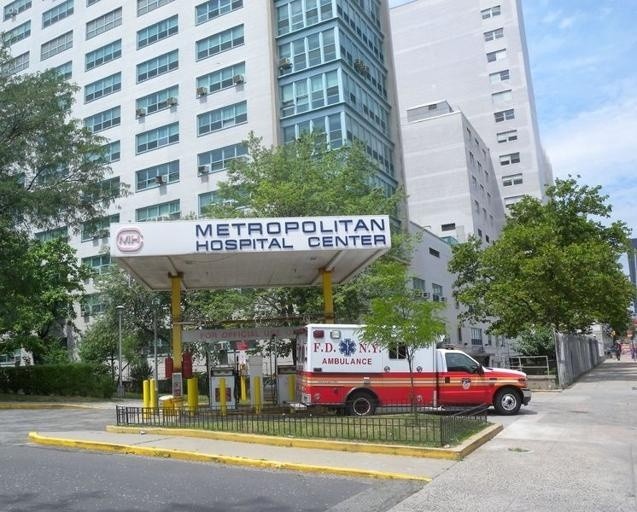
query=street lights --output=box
[115,304,125,397]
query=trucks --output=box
[293,323,531,418]
